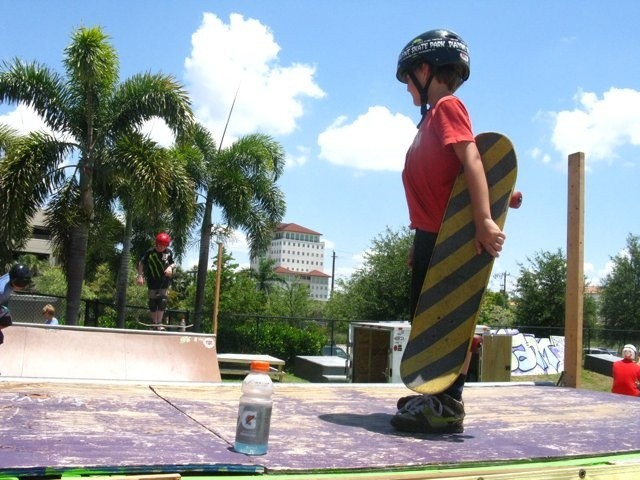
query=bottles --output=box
[233,360,274,458]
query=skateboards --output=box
[138,322,194,331]
[400,132,523,395]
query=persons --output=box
[611,343,639,399]
[389,29,506,435]
[40,302,58,324]
[136,233,178,330]
[0,264,34,345]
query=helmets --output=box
[156,233,170,247]
[622,344,636,359]
[10,264,30,286]
[396,30,469,84]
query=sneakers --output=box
[157,322,165,330]
[390,393,464,433]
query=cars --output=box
[322,344,350,359]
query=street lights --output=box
[213,231,225,334]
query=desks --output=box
[217,353,285,382]
